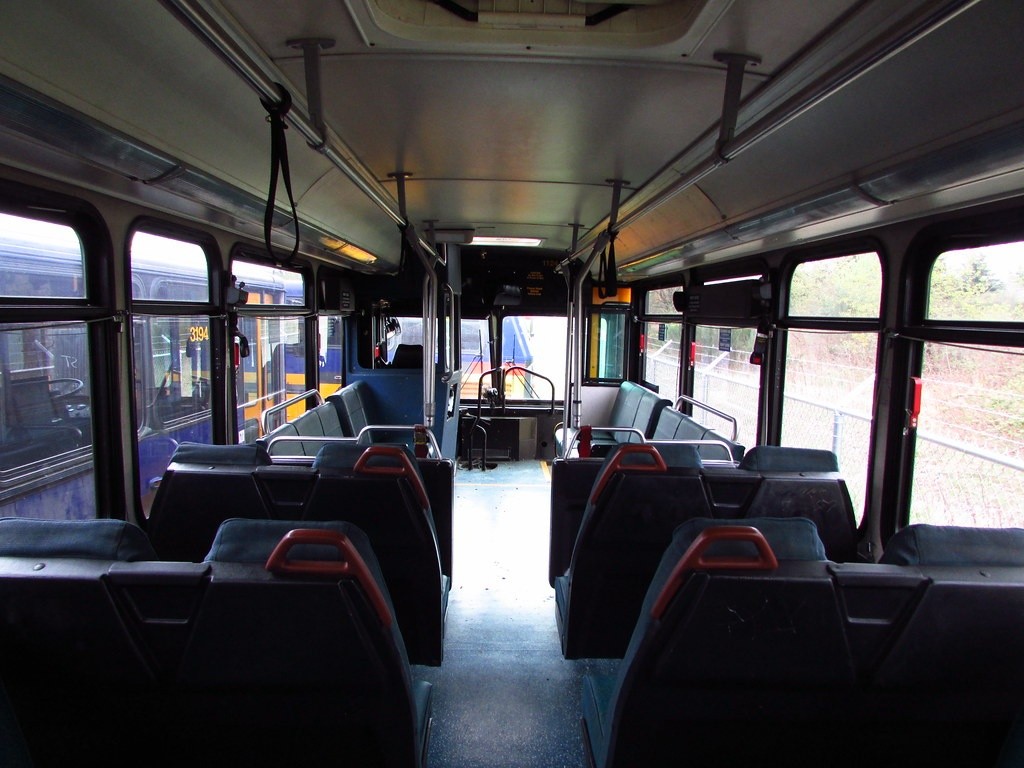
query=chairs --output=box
[390,344,424,369]
[0,439,1024,767]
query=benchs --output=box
[254,380,407,466]
[555,381,746,462]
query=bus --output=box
[392,302,537,402]
[188,276,408,438]
[1,239,289,532]
